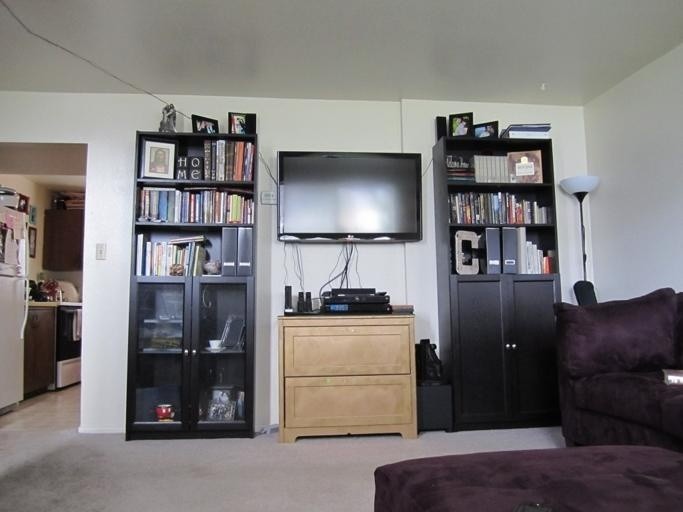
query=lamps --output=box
[559,176,600,308]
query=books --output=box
[133,232,206,277]
[516,226,554,275]
[444,149,544,185]
[447,190,549,224]
[661,368,683,387]
[202,140,255,183]
[139,186,255,225]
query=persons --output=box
[149,150,168,174]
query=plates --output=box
[206,347,226,352]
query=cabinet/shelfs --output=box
[279,307,417,442]
[42,208,83,271]
[25,300,57,396]
[433,137,561,430]
[124,131,257,437]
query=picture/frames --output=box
[28,226,37,258]
[19,194,30,213]
[228,112,257,134]
[192,114,219,133]
[474,121,499,137]
[448,112,472,136]
[141,140,175,179]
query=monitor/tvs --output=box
[275,151,423,244]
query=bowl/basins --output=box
[155,404,175,419]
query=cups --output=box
[210,340,222,347]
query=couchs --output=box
[373,442,681,512]
[554,288,683,453]
